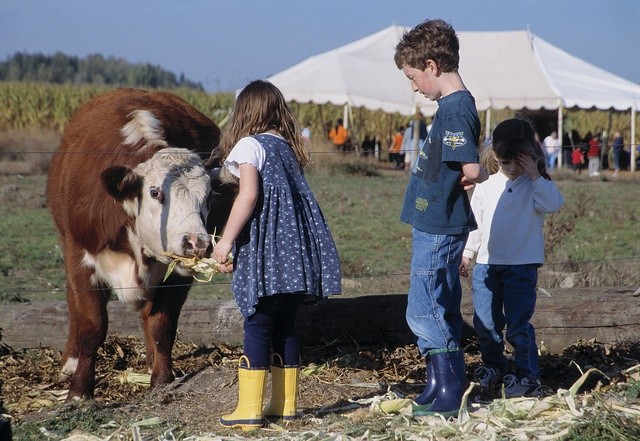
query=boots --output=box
[417,349,472,418]
[220,354,269,431]
[263,352,301,420]
[414,352,438,405]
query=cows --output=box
[46,87,223,410]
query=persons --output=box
[458,119,564,400]
[543,120,621,176]
[214,80,341,433]
[299,117,434,173]
[394,18,480,421]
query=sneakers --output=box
[496,371,543,397]
[475,365,503,404]
